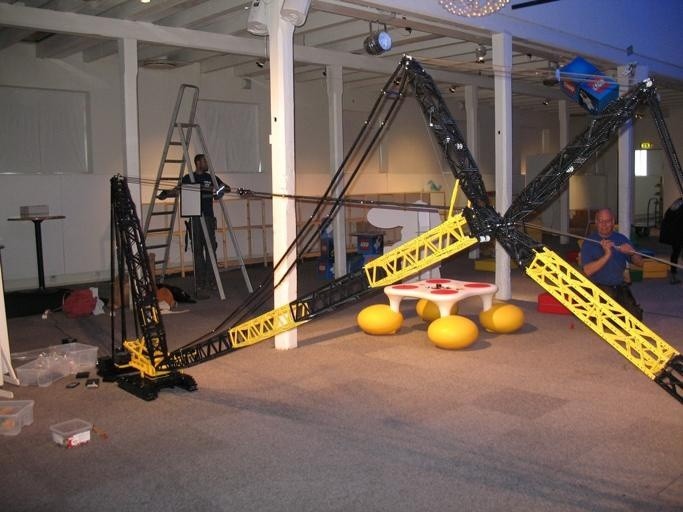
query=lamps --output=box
[439,0,509,18]
[255,57,268,69]
[364,13,392,57]
[475,43,489,65]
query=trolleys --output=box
[631,198,657,237]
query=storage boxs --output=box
[48,342,99,374]
[20,205,49,216]
[48,418,93,450]
[16,356,70,388]
[0,399,34,436]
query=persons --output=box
[579,206,643,331]
[657,196,682,285]
[156,152,231,293]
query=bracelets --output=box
[629,249,635,257]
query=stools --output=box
[426,313,480,351]
[416,299,459,322]
[356,302,405,336]
[479,303,526,333]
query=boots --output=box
[196,281,218,290]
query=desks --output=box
[7,215,67,300]
[383,277,498,317]
